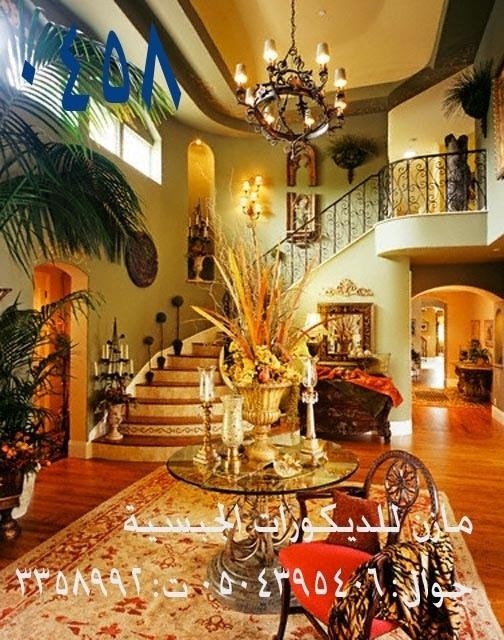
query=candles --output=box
[93,343,135,376]
[188,214,209,238]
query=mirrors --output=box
[317,301,375,367]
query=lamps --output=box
[233,0,348,162]
[238,175,264,249]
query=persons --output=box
[443,133,476,213]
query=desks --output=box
[164,440,360,615]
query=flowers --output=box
[0,431,52,479]
[181,232,345,389]
[184,240,214,263]
[90,380,138,426]
[329,314,362,343]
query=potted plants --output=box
[440,57,494,140]
[0,288,108,520]
[321,134,378,183]
[142,296,184,385]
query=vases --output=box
[0,468,26,498]
[230,379,294,463]
[338,343,352,352]
[105,403,126,440]
[191,256,206,281]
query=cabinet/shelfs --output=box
[298,372,394,443]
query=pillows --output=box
[326,488,385,556]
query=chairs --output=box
[271,447,456,639]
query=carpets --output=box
[1,460,504,639]
